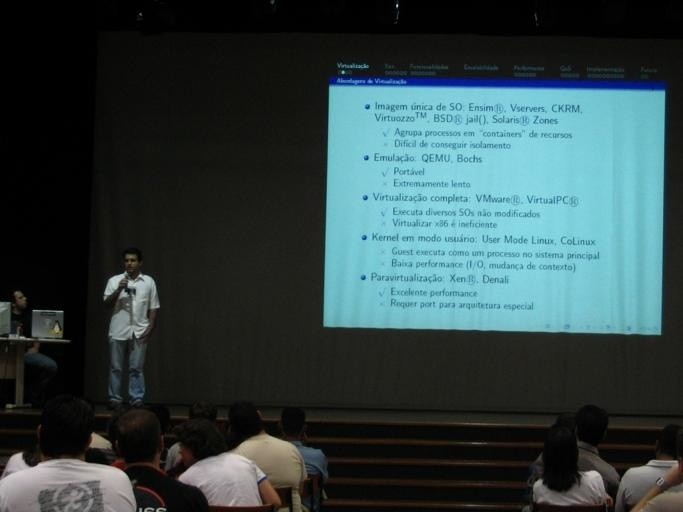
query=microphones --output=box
[124,273,129,292]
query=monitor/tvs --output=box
[0,302,10,337]
[31,309,64,340]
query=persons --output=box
[526,402,683,511]
[0,400,330,511]
[9,286,73,411]
[103,248,163,413]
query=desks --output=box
[0,336,72,411]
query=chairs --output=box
[132,476,324,512]
